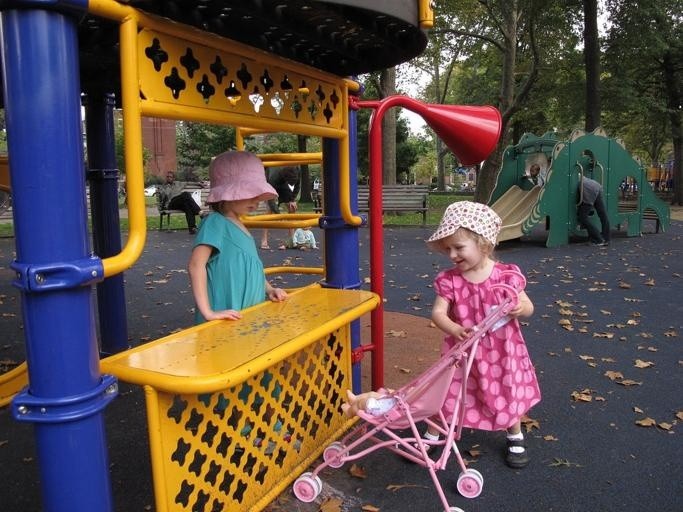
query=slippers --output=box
[260,244,270,249]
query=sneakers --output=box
[278,241,287,250]
[200,213,208,218]
[283,433,301,451]
[190,228,198,234]
[592,240,607,246]
[235,434,276,453]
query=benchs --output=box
[155,182,268,232]
[617,201,670,233]
[313,184,429,228]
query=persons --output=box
[413,200,542,468]
[278,225,318,251]
[260,166,301,250]
[521,157,611,246]
[160,170,209,234]
[188,151,304,454]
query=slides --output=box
[490,184,546,242]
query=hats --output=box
[426,202,502,249]
[204,151,279,205]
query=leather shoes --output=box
[403,437,436,462]
[505,439,529,467]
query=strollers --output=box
[292,270,528,511]
